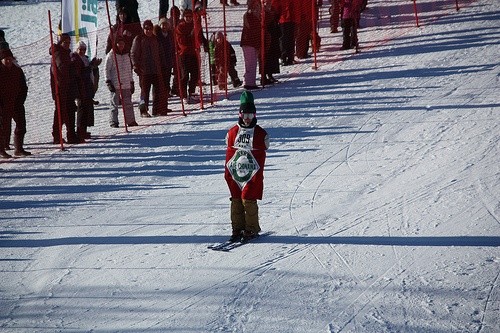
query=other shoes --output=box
[230,232,244,242]
[67,133,86,144]
[261,79,271,85]
[234,81,242,87]
[14,149,32,156]
[141,91,200,117]
[0,151,12,158]
[245,85,260,90]
[53,133,66,144]
[79,134,91,139]
[129,123,139,126]
[340,46,350,50]
[241,232,258,242]
[111,124,119,128]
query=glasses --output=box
[243,113,254,120]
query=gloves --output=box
[130,81,134,94]
[106,82,115,92]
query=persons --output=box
[169,6,181,97]
[328,0,368,50]
[130,20,162,118]
[220,0,240,6]
[225,91,269,243]
[176,8,201,104]
[104,35,138,128]
[0,28,31,158]
[209,32,243,88]
[159,0,169,20]
[240,0,319,90]
[153,18,172,116]
[106,7,142,105]
[115,0,140,24]
[181,0,207,46]
[49,33,102,144]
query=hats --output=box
[58,34,70,42]
[0,48,13,60]
[159,18,170,26]
[239,91,256,112]
[77,41,87,48]
[183,9,193,16]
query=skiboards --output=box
[215,228,275,251]
